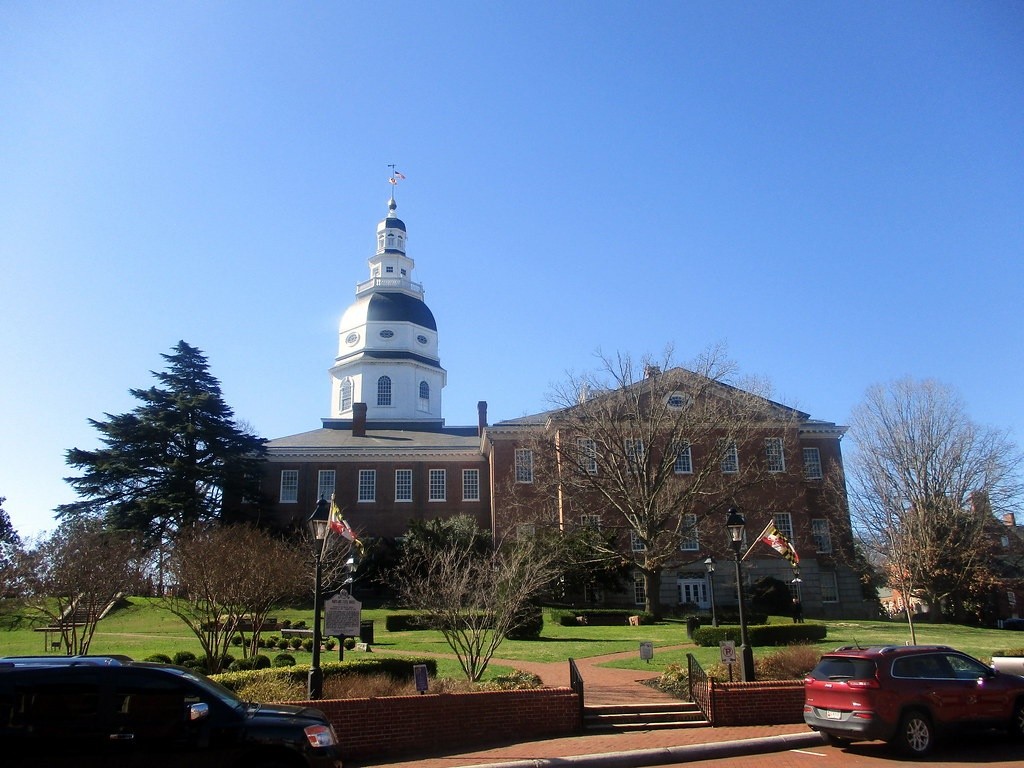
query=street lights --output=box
[344,553,363,594]
[725,508,756,683]
[305,494,332,700]
[703,554,718,628]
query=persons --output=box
[791,595,802,624]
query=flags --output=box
[329,502,364,555]
[760,523,798,568]
[388,171,406,187]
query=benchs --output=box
[281,628,314,638]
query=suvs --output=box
[802,644,1024,758]
[0,654,344,768]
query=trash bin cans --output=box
[686,616,701,637]
[360,620,375,643]
